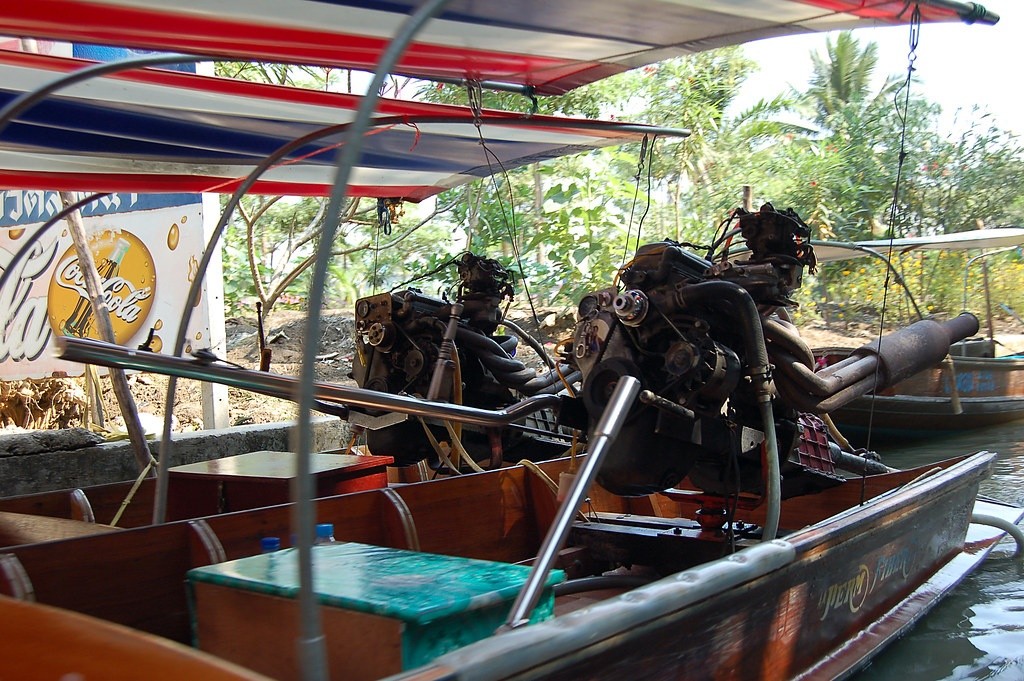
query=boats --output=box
[0,0,1024,681]
[712,228,1024,433]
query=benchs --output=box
[0,512,124,546]
[168,451,394,517]
[183,541,566,681]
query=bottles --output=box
[260,537,280,553]
[314,524,336,544]
[62,237,131,339]
